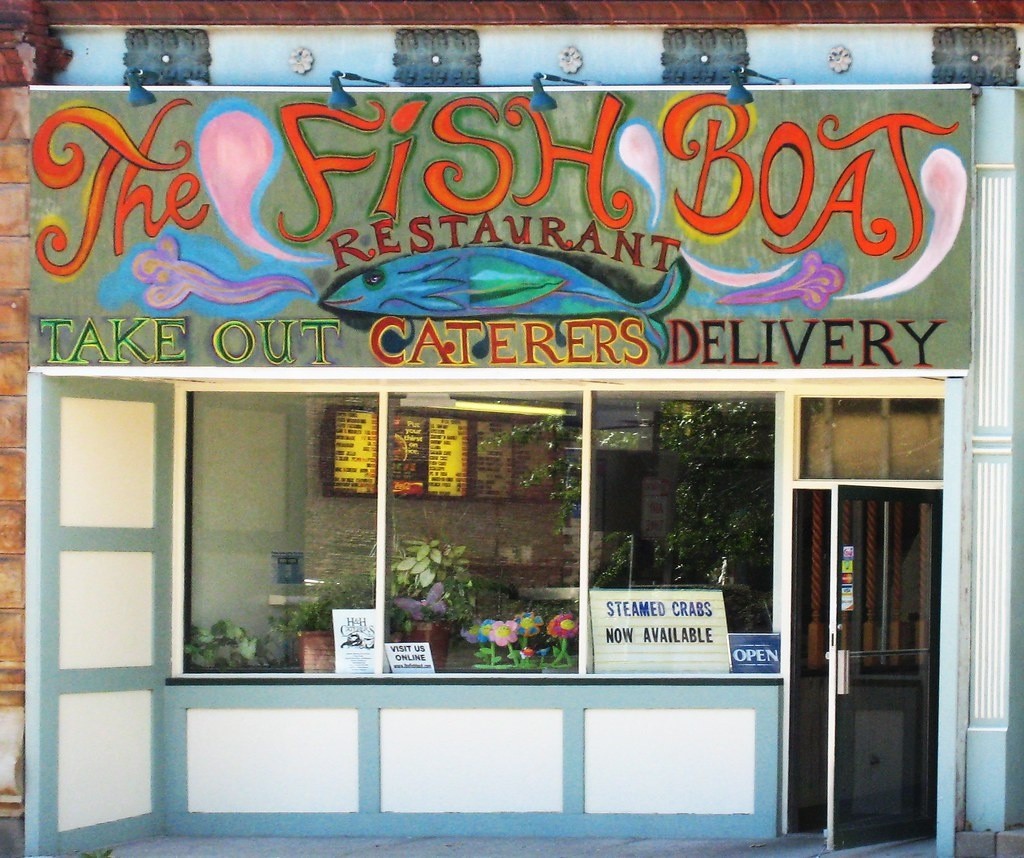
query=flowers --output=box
[461,610,578,669]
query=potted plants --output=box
[268,587,352,674]
[386,535,480,668]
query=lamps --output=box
[124,69,201,108]
[329,69,401,110]
[399,392,576,416]
[530,73,601,111]
[727,65,797,104]
